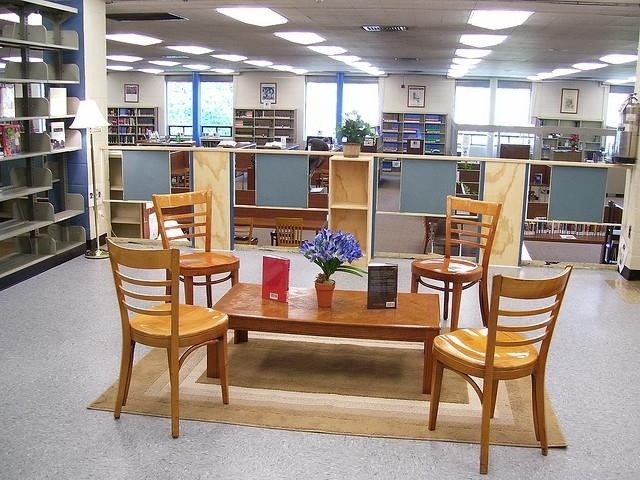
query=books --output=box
[109,109,151,144]
[235,120,253,128]
[367,261,399,310]
[523,218,606,242]
[265,141,285,149]
[602,224,620,264]
[218,140,236,147]
[260,252,292,305]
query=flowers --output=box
[297,226,369,282]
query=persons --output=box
[3,125,22,157]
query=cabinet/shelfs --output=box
[0,0,87,292]
[106,149,146,241]
[379,111,448,154]
[233,108,297,146]
[532,117,604,161]
[107,105,159,146]
[327,156,374,269]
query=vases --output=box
[315,280,337,309]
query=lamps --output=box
[68,99,112,260]
[407,85,425,108]
[260,82,277,104]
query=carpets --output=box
[87,329,568,451]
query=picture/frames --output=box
[560,88,579,114]
[124,83,140,102]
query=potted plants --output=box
[334,110,374,157]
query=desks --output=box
[205,282,447,394]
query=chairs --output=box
[230,215,303,247]
[106,187,242,439]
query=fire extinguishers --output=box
[617,90,640,164]
